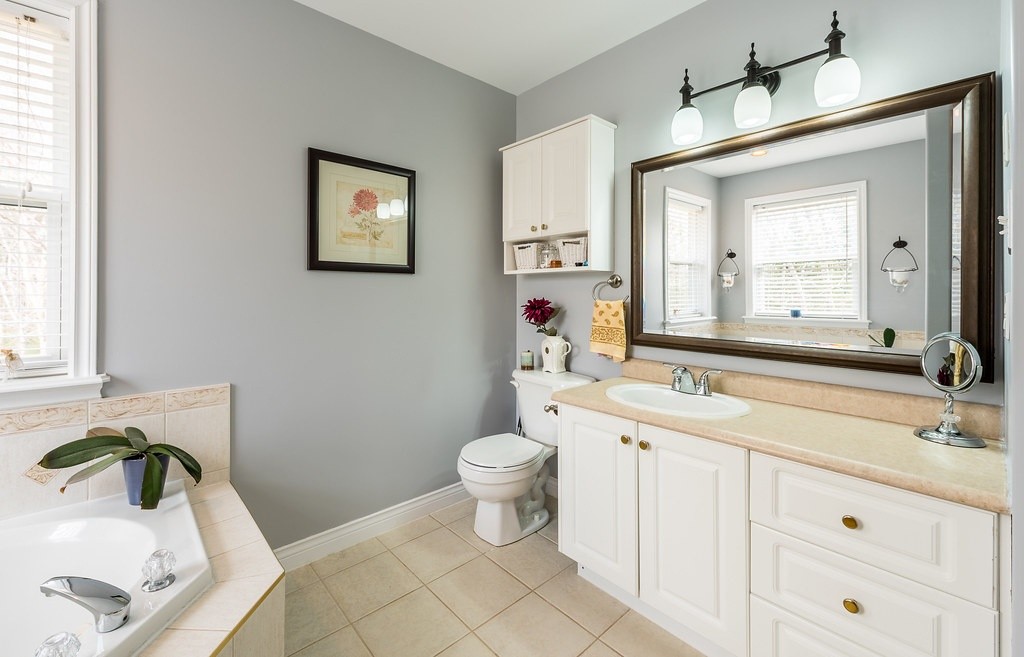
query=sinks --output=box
[615,385,739,413]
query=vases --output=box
[542,336,572,374]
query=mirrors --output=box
[914,332,986,448]
[717,249,739,288]
[630,71,1003,389]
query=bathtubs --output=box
[0,519,150,657]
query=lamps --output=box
[734,42,781,128]
[813,11,860,107]
[880,235,919,293]
[671,69,703,145]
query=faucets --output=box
[662,362,724,397]
[40,576,131,632]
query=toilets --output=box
[457,364,598,547]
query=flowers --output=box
[520,297,560,335]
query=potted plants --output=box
[37,426,202,510]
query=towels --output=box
[720,272,736,289]
[887,267,910,289]
[589,298,628,364]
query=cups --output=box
[540,248,558,269]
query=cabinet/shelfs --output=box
[497,114,617,275]
[557,403,1012,657]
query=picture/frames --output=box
[307,147,417,275]
